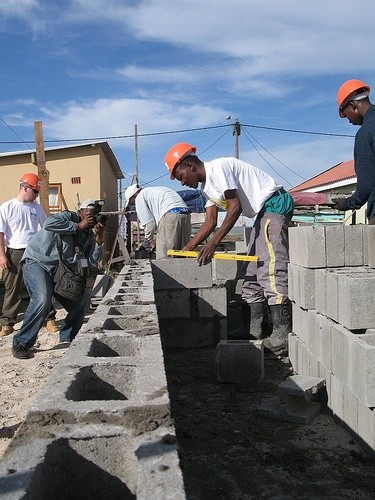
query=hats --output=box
[77,199,102,212]
[124,184,143,208]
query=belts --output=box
[266,188,287,202]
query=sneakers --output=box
[12,345,29,358]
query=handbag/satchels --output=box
[53,255,87,302]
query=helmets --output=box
[164,142,196,180]
[337,81,370,119]
[19,173,41,191]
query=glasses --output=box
[30,188,39,194]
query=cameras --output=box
[87,200,107,227]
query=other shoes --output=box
[0,326,14,337]
[47,320,59,332]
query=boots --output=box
[228,302,273,340]
[261,302,292,352]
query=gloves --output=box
[327,195,356,211]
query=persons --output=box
[12,199,105,359]
[1,173,57,337]
[124,184,192,260]
[330,79,375,225]
[164,142,294,352]
